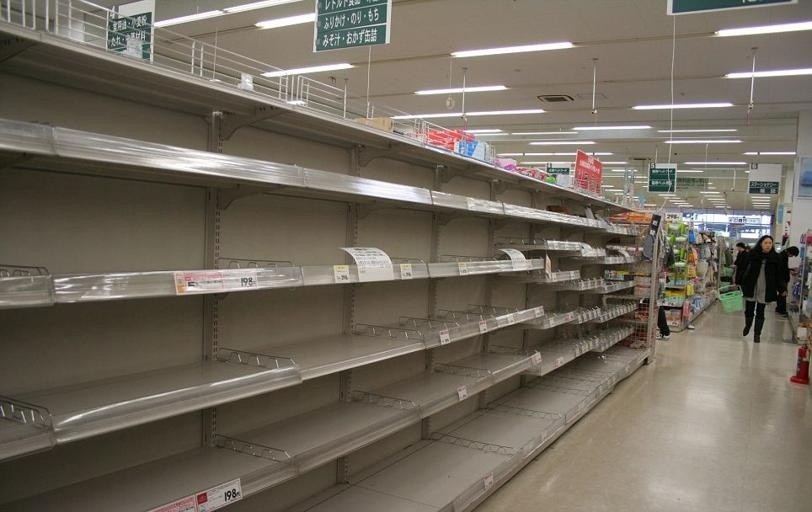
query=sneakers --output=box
[655,332,671,341]
[753,333,761,343]
[742,327,750,337]
[775,310,789,320]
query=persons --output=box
[657,307,670,341]
[732,243,748,284]
[743,245,751,253]
[776,245,799,319]
[740,234,782,343]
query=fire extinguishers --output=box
[796,343,810,384]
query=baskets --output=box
[717,285,745,314]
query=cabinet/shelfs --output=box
[0,4,545,512]
[542,172,718,447]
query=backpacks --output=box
[664,238,675,268]
